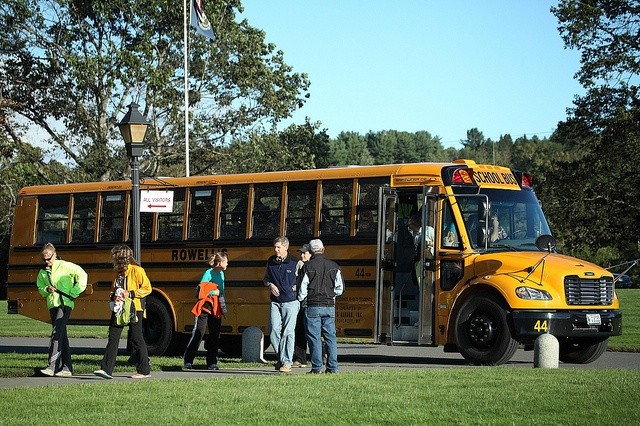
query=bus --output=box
[6,158,623,366]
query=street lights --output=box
[118,100,151,266]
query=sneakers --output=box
[208,364,219,371]
[55,367,72,377]
[324,369,337,375]
[279,363,292,373]
[131,372,151,379]
[292,359,307,368]
[184,362,192,370]
[94,370,113,380]
[273,362,283,370]
[306,369,322,374]
[40,368,54,377]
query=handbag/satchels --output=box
[116,298,136,326]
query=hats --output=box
[297,244,309,253]
[309,239,324,250]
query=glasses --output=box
[42,254,54,261]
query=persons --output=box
[263,236,300,372]
[298,238,343,374]
[393,218,414,294]
[36,243,88,377]
[292,243,328,368]
[93,244,152,379]
[182,252,228,373]
[189,188,378,225]
[408,216,435,344]
[477,214,510,247]
